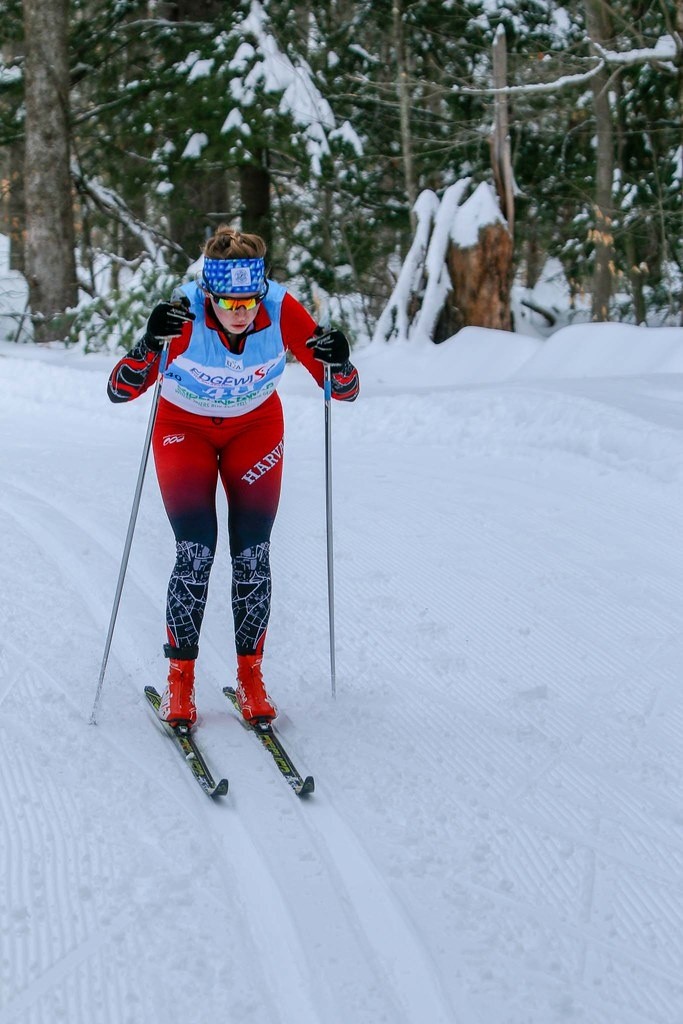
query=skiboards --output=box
[143,686,315,797]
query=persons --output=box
[107,224,360,727]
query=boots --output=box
[158,644,199,728]
[236,654,279,725]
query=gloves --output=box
[306,325,350,374]
[144,296,195,352]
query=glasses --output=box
[209,293,265,311]
